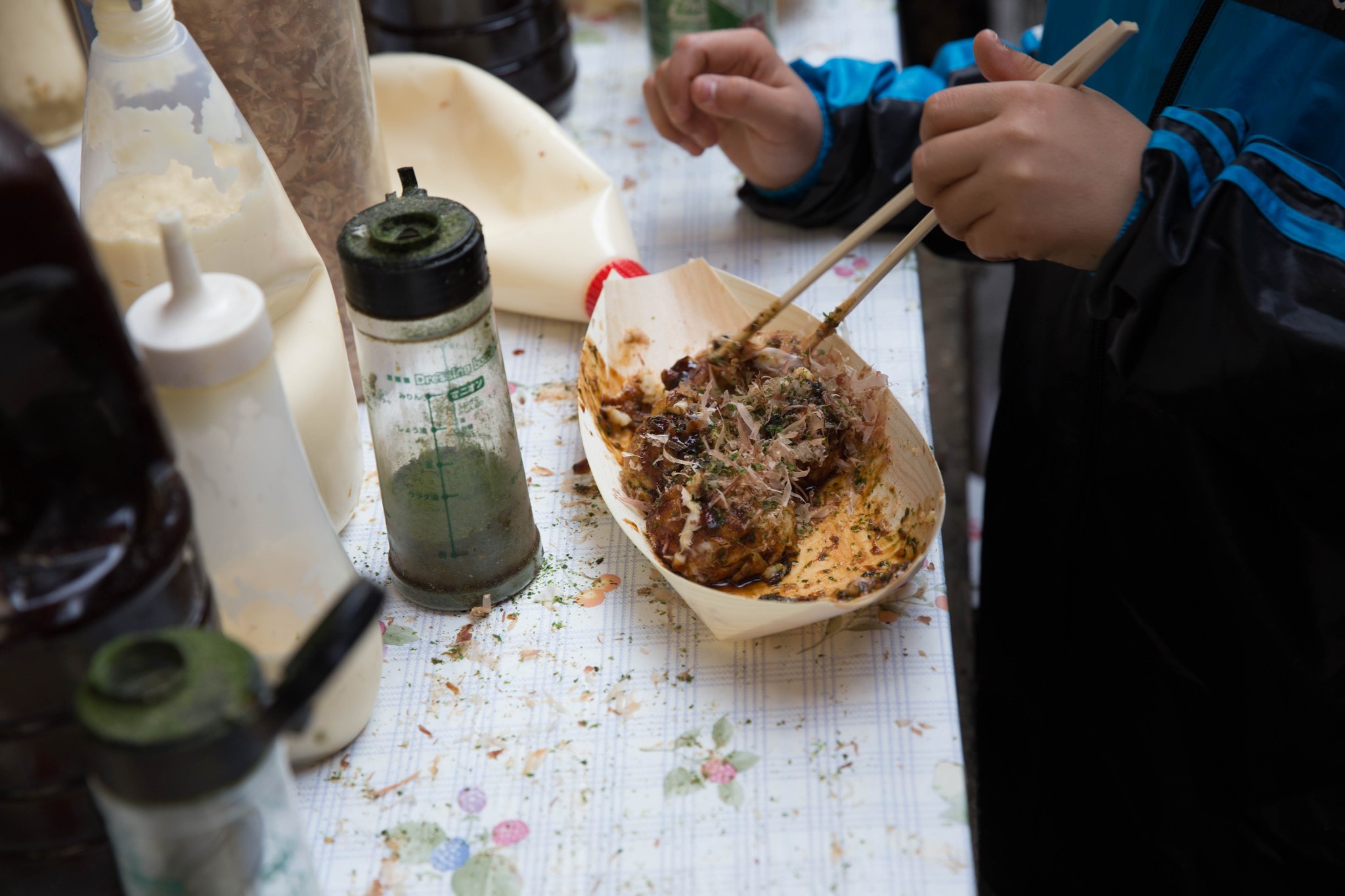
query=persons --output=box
[644,0,1345,895]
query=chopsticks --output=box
[710,17,1140,360]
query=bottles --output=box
[73,627,325,896]
[368,50,652,323]
[335,165,545,613]
[79,0,365,538]
[125,212,385,766]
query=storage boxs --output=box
[579,255,944,642]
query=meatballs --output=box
[621,340,845,586]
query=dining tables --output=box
[41,0,977,896]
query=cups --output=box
[0,0,89,148]
[360,0,579,119]
[638,0,779,72]
